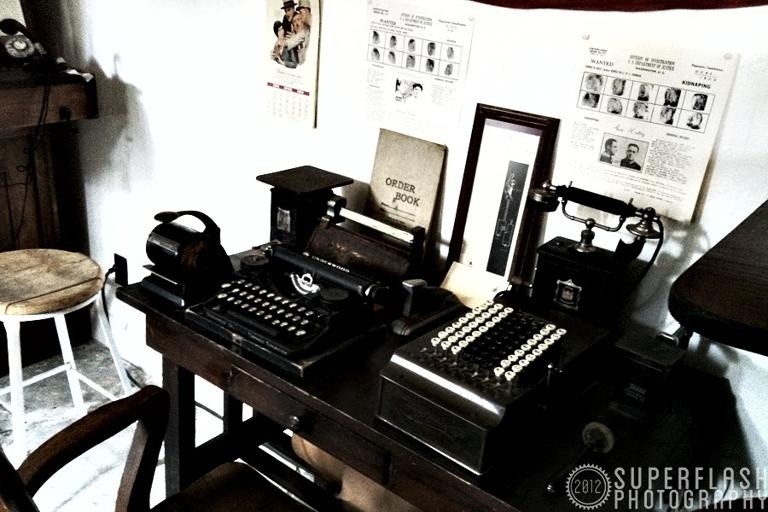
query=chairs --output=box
[2,385,310,511]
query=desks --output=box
[117,242,761,511]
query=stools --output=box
[2,248,138,461]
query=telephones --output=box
[0,18,48,81]
[530,179,664,326]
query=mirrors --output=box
[444,102,561,290]
